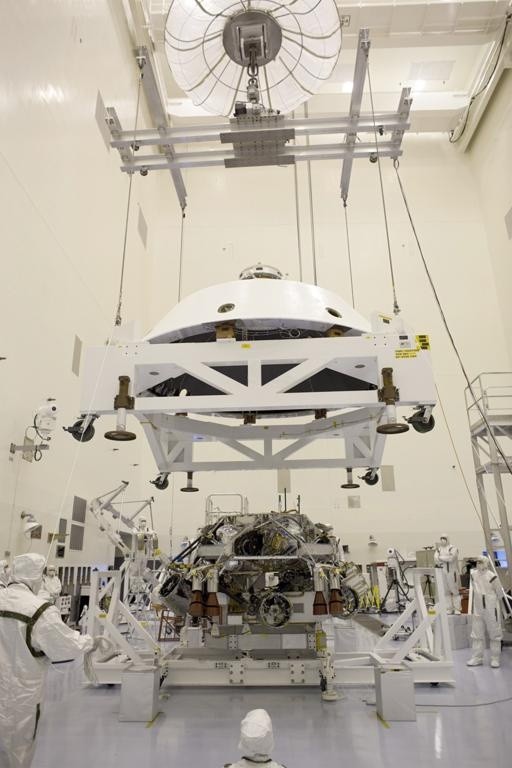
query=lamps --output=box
[21,511,40,534]
[368,535,377,546]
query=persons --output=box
[39,564,65,605]
[433,531,467,617]
[1,553,97,767]
[131,515,155,578]
[225,705,288,768]
[464,554,502,667]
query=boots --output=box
[446,596,461,615]
[467,639,484,666]
[490,640,502,668]
